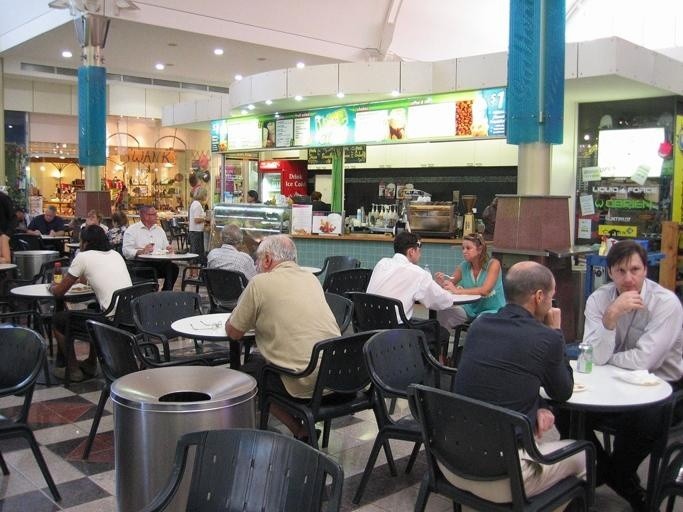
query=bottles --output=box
[370,202,398,226]
[423,264,430,275]
[52,262,63,285]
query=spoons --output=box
[443,274,455,280]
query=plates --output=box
[572,380,586,392]
[619,375,659,386]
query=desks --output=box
[415,291,484,321]
[170,311,255,366]
[538,358,674,454]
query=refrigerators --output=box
[257,158,309,200]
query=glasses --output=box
[146,211,158,216]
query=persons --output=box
[46,224,133,370]
[0,188,129,259]
[310,192,327,211]
[226,233,358,449]
[188,187,257,309]
[364,231,452,367]
[436,233,506,336]
[435,262,598,512]
[246,190,261,203]
[551,240,683,512]
[122,205,179,291]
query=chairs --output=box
[129,290,231,366]
[63,281,160,388]
[350,291,441,361]
[1,323,62,506]
[146,427,349,511]
[352,328,457,506]
[312,255,363,292]
[82,317,208,460]
[405,383,596,511]
[0,216,212,322]
[322,292,355,334]
[594,383,683,511]
[205,266,248,316]
[326,268,374,299]
[447,322,473,369]
[259,328,400,478]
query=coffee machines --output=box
[460,194,478,236]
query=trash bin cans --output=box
[14,251,60,281]
[110,365,259,512]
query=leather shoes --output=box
[628,479,653,511]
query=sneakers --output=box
[49,359,98,383]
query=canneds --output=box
[576,343,593,375]
